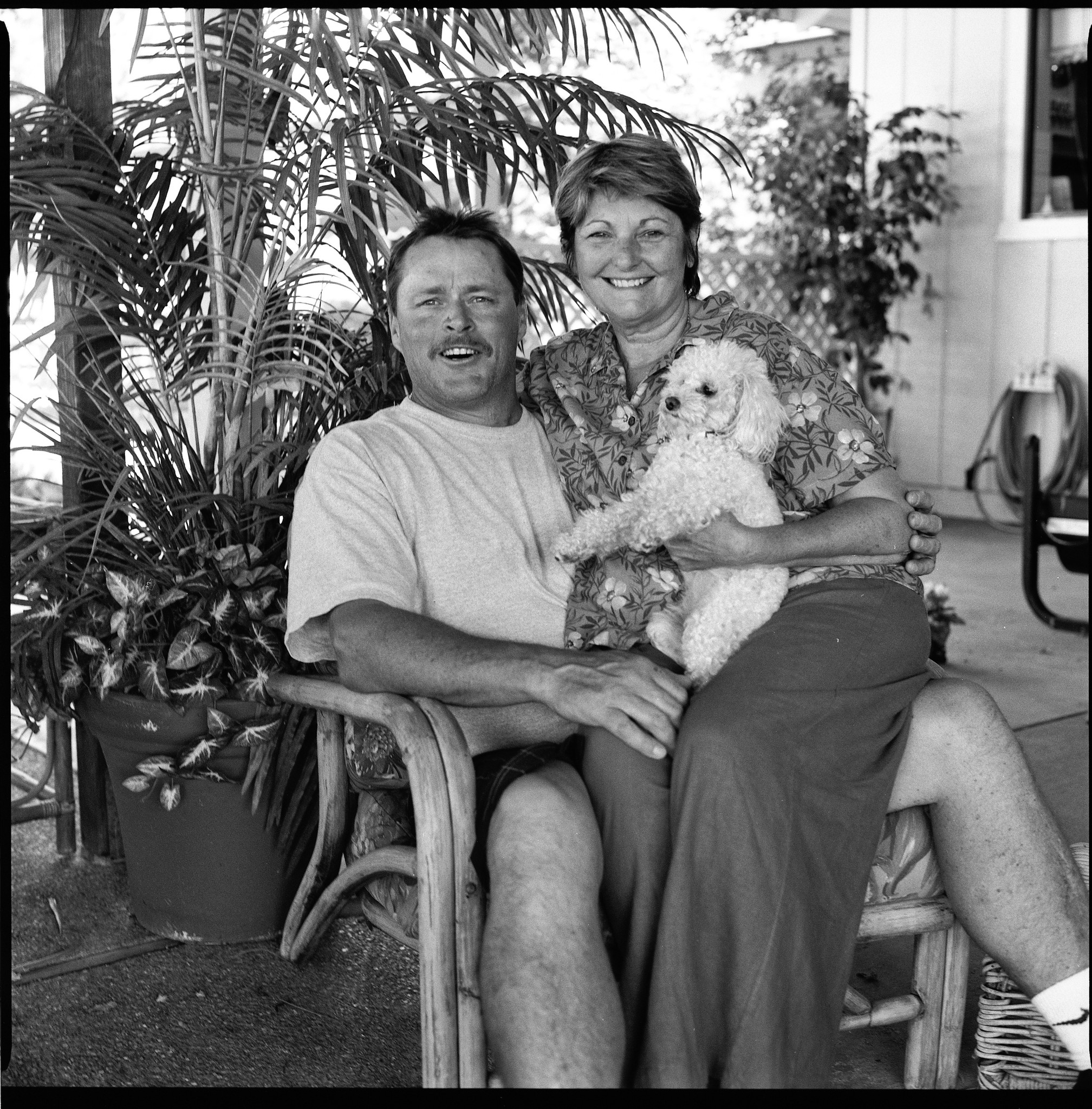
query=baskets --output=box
[973,842,1091,1090]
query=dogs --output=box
[554,336,791,690]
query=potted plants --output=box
[9,5,758,951]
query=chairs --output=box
[262,673,970,1091]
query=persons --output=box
[289,210,1087,1091]
[518,135,933,1090]
